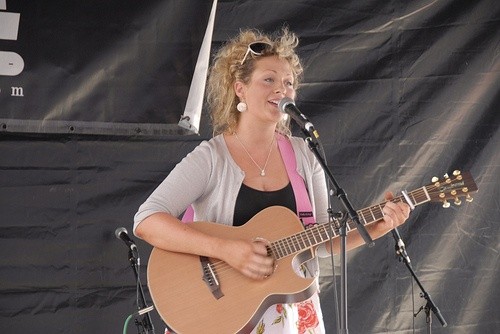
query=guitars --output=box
[147,168,479,334]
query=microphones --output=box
[115,227,136,251]
[279,96,314,132]
[391,227,405,249]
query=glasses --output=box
[241,41,272,65]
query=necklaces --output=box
[232,130,276,177]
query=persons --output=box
[133,29,411,334]
